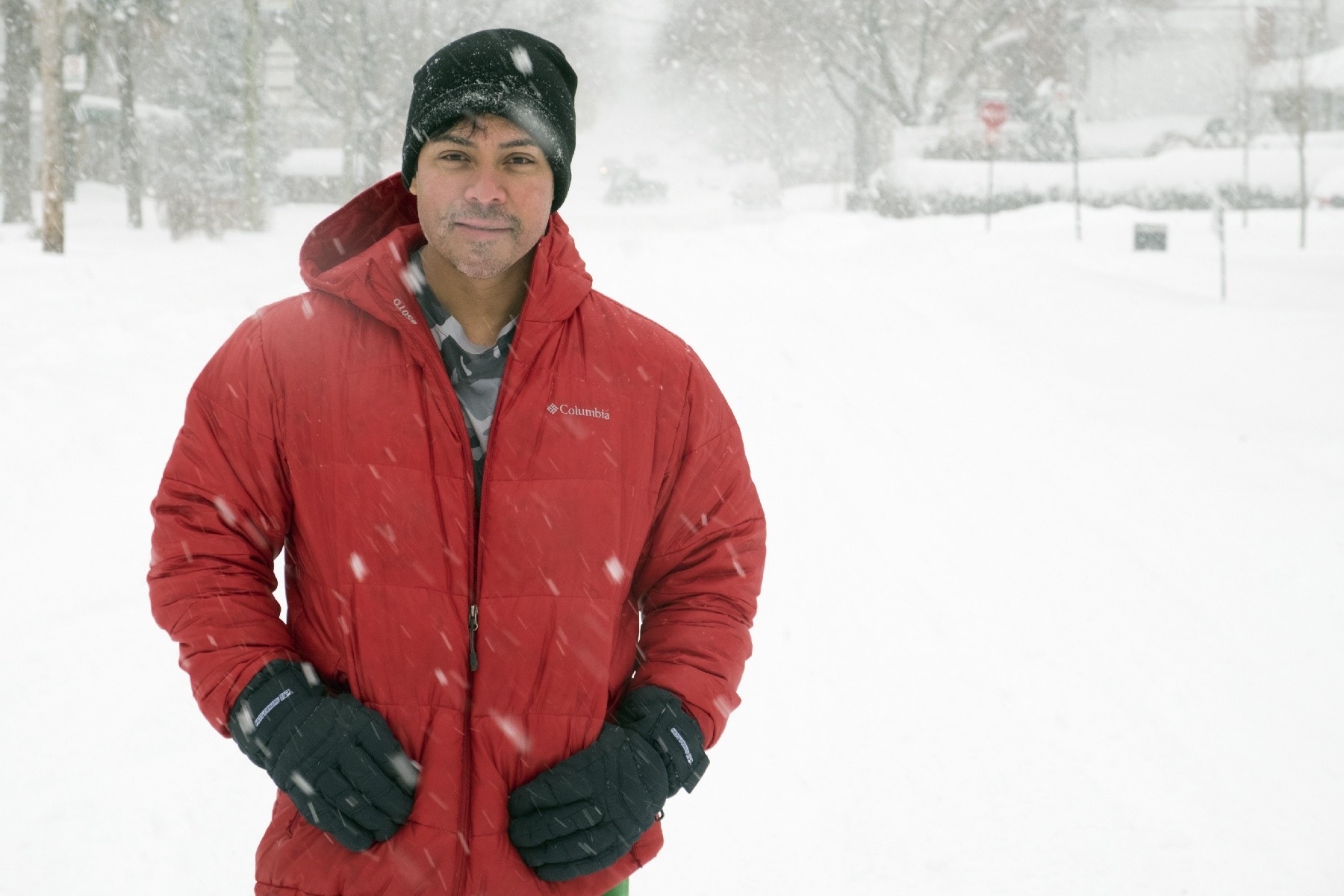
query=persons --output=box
[147,27,766,896]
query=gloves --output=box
[225,656,423,853]
[506,682,711,884]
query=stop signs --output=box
[981,101,1007,128]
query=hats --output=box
[401,28,579,215]
